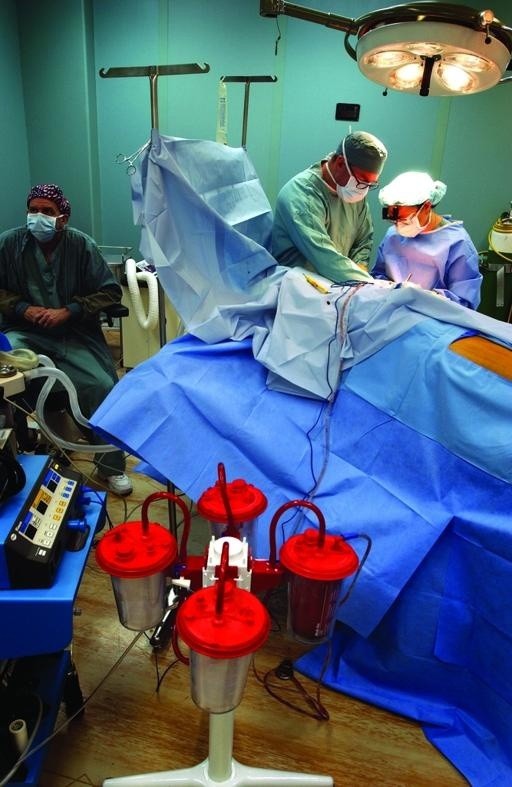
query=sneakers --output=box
[97,468,133,495]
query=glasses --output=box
[349,163,380,190]
[389,208,419,225]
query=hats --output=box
[379,171,447,207]
[27,184,71,217]
[336,132,387,174]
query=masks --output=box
[394,205,432,238]
[26,213,64,242]
[328,156,369,204]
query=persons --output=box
[0,180,138,501]
[370,167,484,313]
[262,129,388,286]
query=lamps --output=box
[258,0,511,97]
